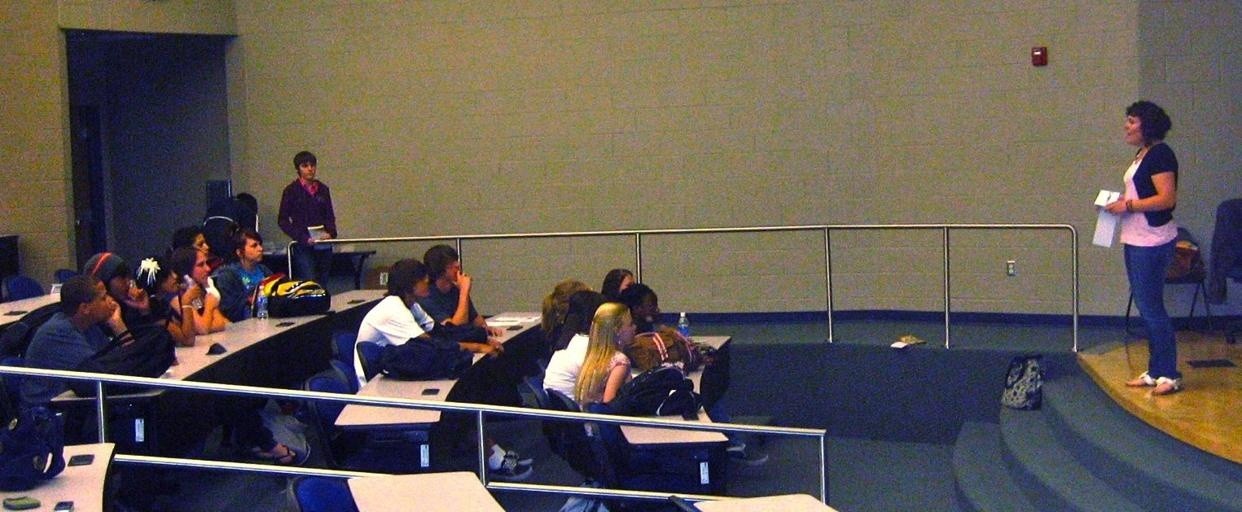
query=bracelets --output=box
[1125,200,1133,212]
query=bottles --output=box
[256,284,268,321]
[677,310,690,340]
[184,274,204,310]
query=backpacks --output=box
[376,337,475,381]
[0,405,67,492]
[68,318,176,399]
[250,273,332,319]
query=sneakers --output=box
[487,451,534,482]
[725,442,769,466]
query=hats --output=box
[80,250,126,286]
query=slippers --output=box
[1126,370,1157,387]
[241,444,298,467]
[1151,375,1185,396]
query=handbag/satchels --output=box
[604,366,705,421]
[623,327,704,374]
[1000,354,1045,411]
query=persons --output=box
[1097,99,1190,397]
[276,150,336,287]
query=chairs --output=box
[1121,228,1216,332]
[2,237,839,510]
